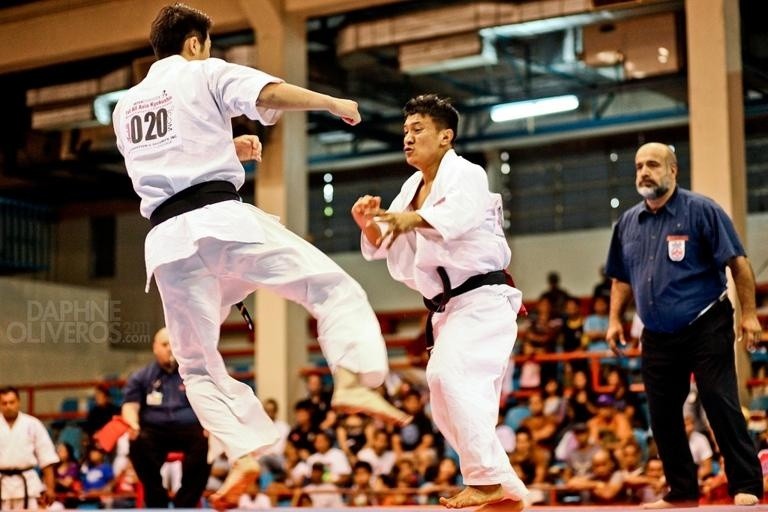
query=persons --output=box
[349,93,530,512]
[111,2,415,508]
[603,142,764,510]
[39,264,768,512]
[0,387,61,512]
